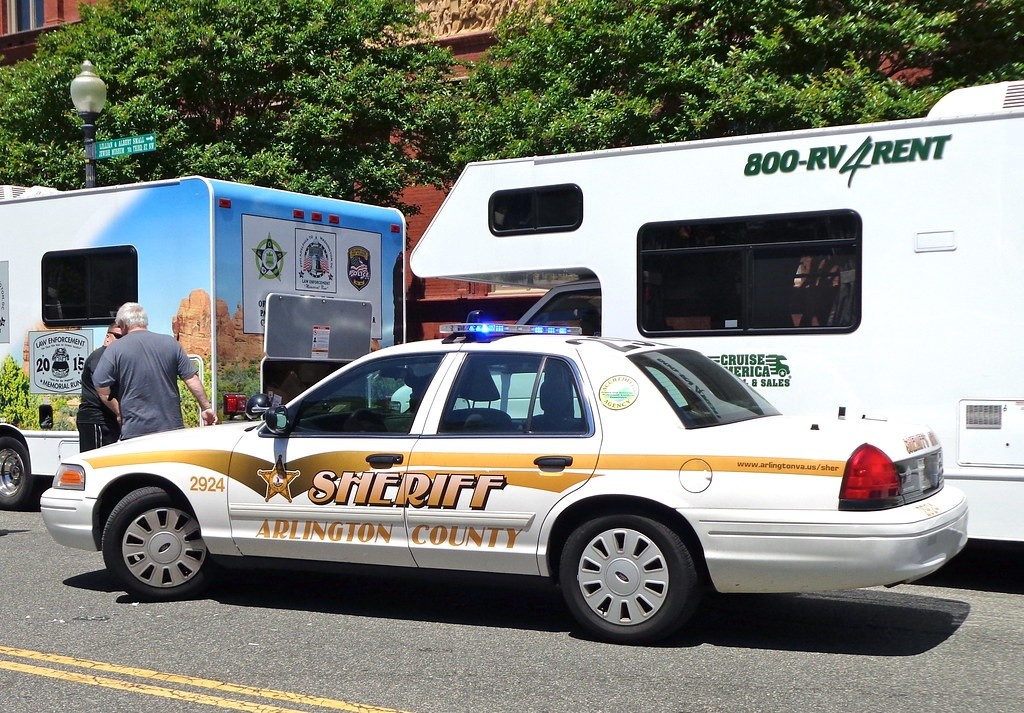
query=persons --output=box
[90,300,218,441]
[354,372,452,436]
[76,322,129,452]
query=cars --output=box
[39,312,972,645]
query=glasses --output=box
[108,331,124,339]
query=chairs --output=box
[362,366,587,437]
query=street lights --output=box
[73,57,108,188]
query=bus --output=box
[0,173,406,513]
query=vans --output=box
[404,74,1024,540]
